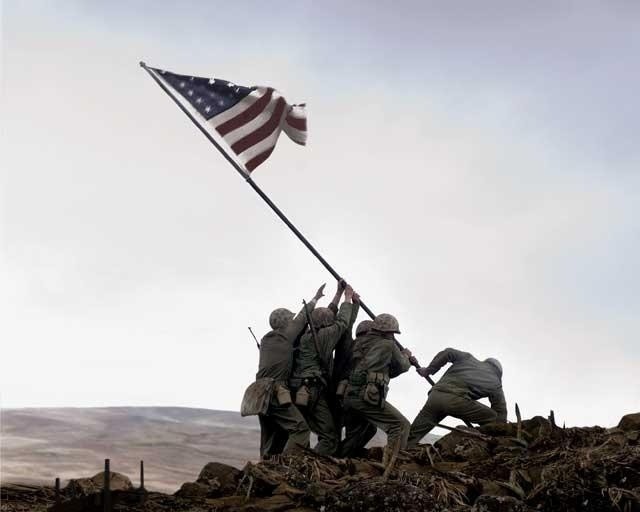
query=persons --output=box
[341,310,413,479]
[249,281,327,463]
[288,276,357,458]
[324,289,377,457]
[404,344,508,454]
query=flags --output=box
[147,65,308,171]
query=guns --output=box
[375,381,388,410]
[248,326,259,351]
[302,299,333,389]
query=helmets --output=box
[310,307,335,328]
[356,319,374,336]
[484,358,502,376]
[268,307,295,330]
[370,313,401,335]
